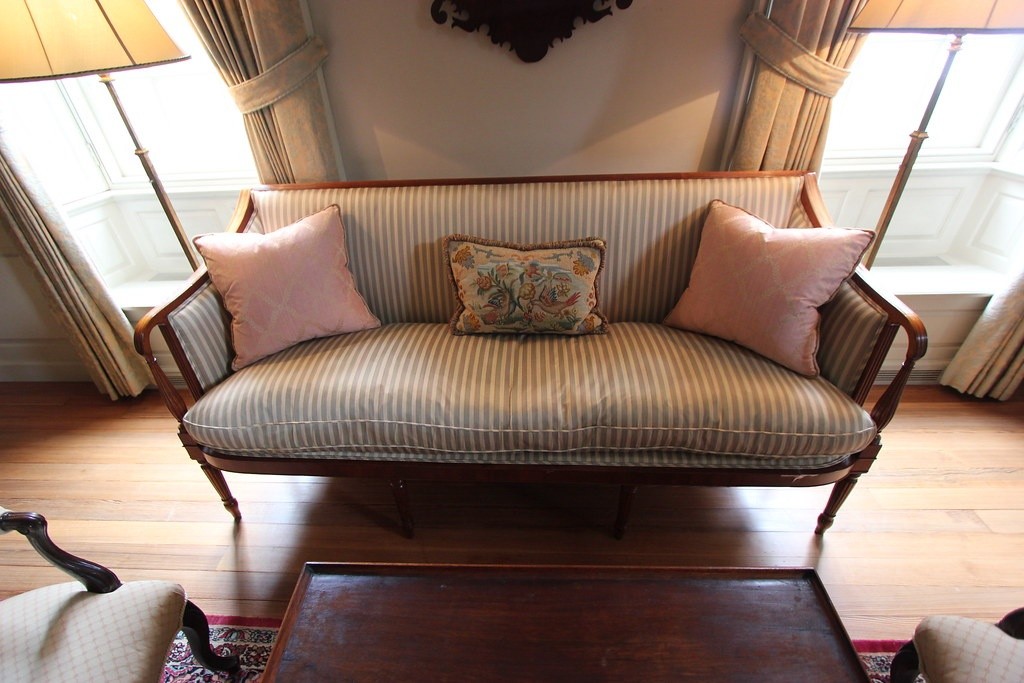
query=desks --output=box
[260,563,873,683]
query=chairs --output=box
[0,506,240,683]
[890,607,1024,683]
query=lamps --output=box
[0,0,200,272]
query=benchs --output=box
[132,170,930,534]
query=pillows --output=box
[442,234,612,336]
[662,198,878,379]
[192,204,382,372]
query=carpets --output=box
[160,614,927,683]
